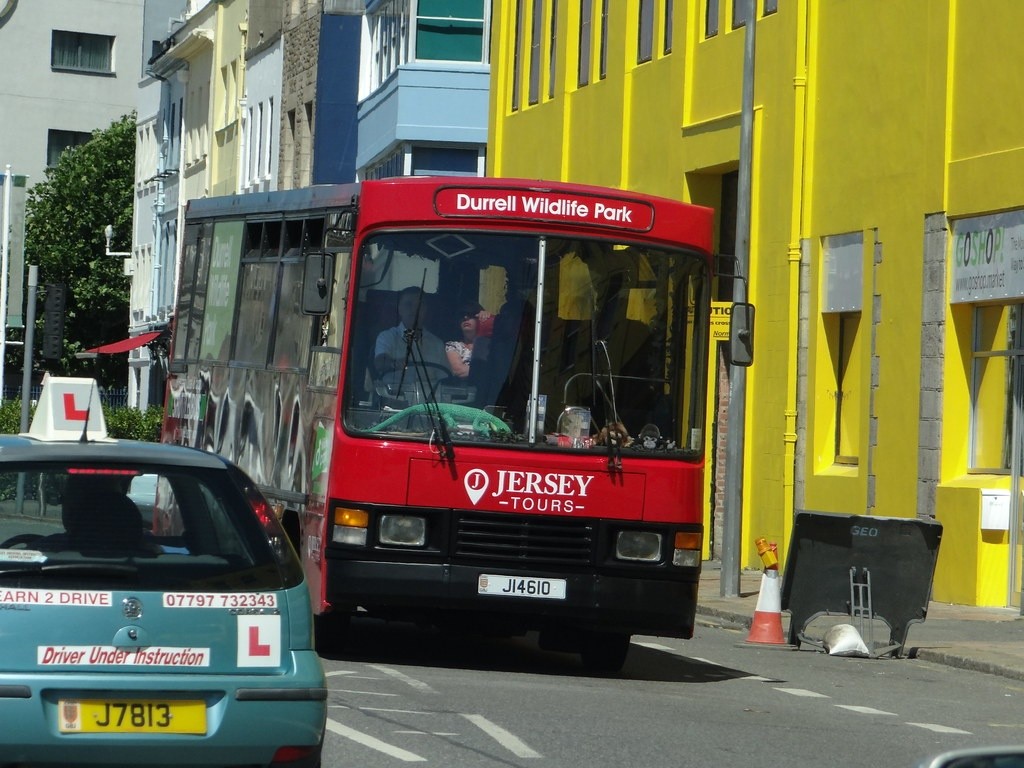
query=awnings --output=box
[86,330,167,353]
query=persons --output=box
[445,303,491,378]
[23,472,162,550]
[367,287,452,402]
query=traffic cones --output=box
[732,539,799,651]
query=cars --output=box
[0,371,330,768]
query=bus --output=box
[145,173,759,677]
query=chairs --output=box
[52,487,145,548]
[363,289,399,402]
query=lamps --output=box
[105,224,133,256]
[144,169,178,184]
[146,68,166,84]
